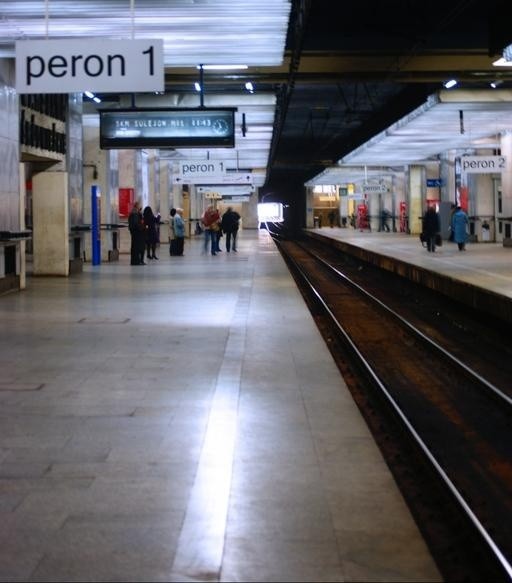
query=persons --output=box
[201,204,241,256]
[328,209,392,233]
[170,207,185,256]
[420,205,442,252]
[128,201,161,266]
[448,204,469,251]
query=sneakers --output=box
[210,249,238,256]
[130,262,146,265]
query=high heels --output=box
[147,255,159,260]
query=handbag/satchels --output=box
[420,233,427,242]
[448,231,454,242]
[436,234,442,246]
